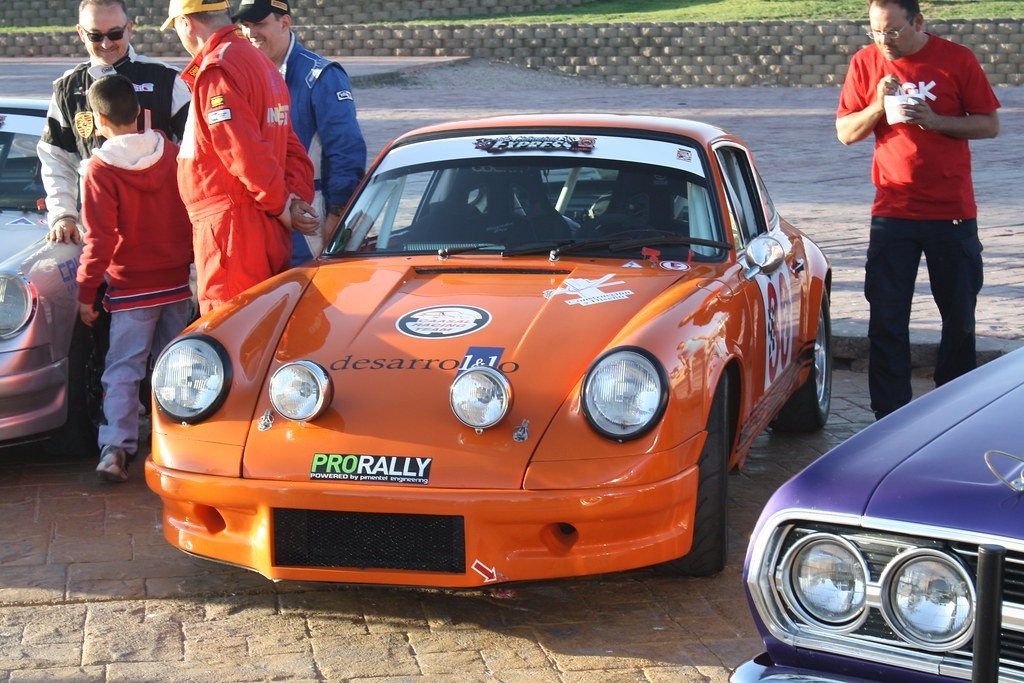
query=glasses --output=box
[866,15,915,40]
[80,22,127,42]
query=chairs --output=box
[595,166,689,235]
[406,199,488,247]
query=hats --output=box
[160,0,230,31]
[231,0,290,24]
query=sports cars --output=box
[0,97,110,449]
[143,113,830,601]
[729,341,1024,683]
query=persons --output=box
[36,0,368,483]
[77,74,195,483]
[835,0,1002,421]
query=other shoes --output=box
[138,401,146,415]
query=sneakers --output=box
[97,445,128,482]
